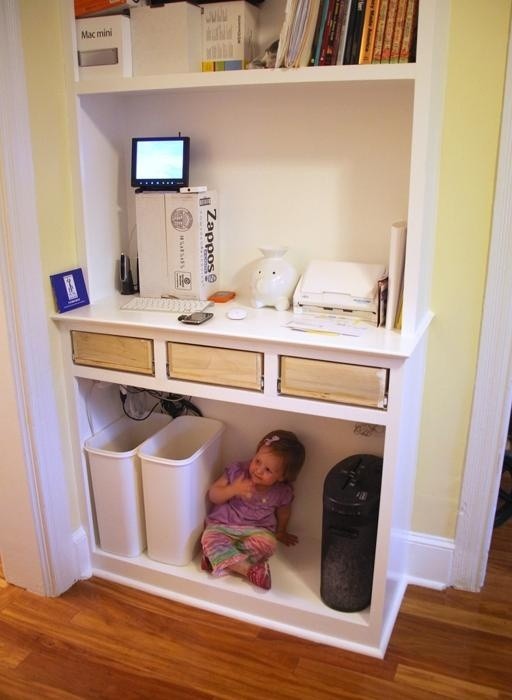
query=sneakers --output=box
[246,560,271,590]
[201,555,212,571]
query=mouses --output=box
[227,308,248,320]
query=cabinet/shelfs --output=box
[17,0,451,662]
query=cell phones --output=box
[182,312,213,324]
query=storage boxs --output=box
[130,2,202,78]
[197,2,258,72]
[75,15,131,79]
[137,189,219,300]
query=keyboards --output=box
[120,297,215,314]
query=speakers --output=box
[119,254,136,295]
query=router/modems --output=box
[180,186,207,193]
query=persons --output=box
[200,430,306,590]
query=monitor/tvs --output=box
[131,132,190,193]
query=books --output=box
[275,0,419,70]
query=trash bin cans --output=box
[320,454,382,612]
